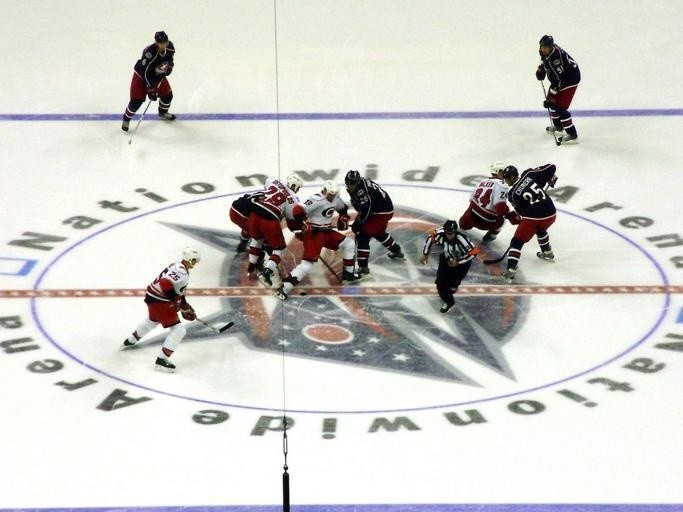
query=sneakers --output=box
[537,251,554,258]
[122,118,129,132]
[159,112,176,120]
[341,274,360,281]
[546,126,563,132]
[440,301,455,313]
[387,252,402,258]
[247,262,288,299]
[237,241,247,250]
[123,339,134,345]
[156,357,175,369]
[355,268,369,276]
[502,269,516,278]
[558,135,573,141]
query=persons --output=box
[503,164,557,279]
[124,247,199,368]
[436,163,522,240]
[122,31,175,131]
[420,220,478,313]
[230,170,404,301]
[536,35,581,141]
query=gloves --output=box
[338,216,349,230]
[508,212,520,224]
[351,220,362,234]
[302,219,311,235]
[146,86,157,101]
[155,67,165,76]
[178,303,196,322]
[543,97,555,107]
[536,65,546,80]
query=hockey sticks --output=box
[129,100,152,144]
[541,79,561,146]
[483,184,550,263]
[195,316,233,333]
[319,256,343,283]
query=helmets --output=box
[286,173,302,191]
[540,35,554,45]
[181,246,200,263]
[443,220,458,233]
[504,166,519,177]
[345,170,360,185]
[490,161,505,175]
[323,180,338,196]
[155,32,168,44]
